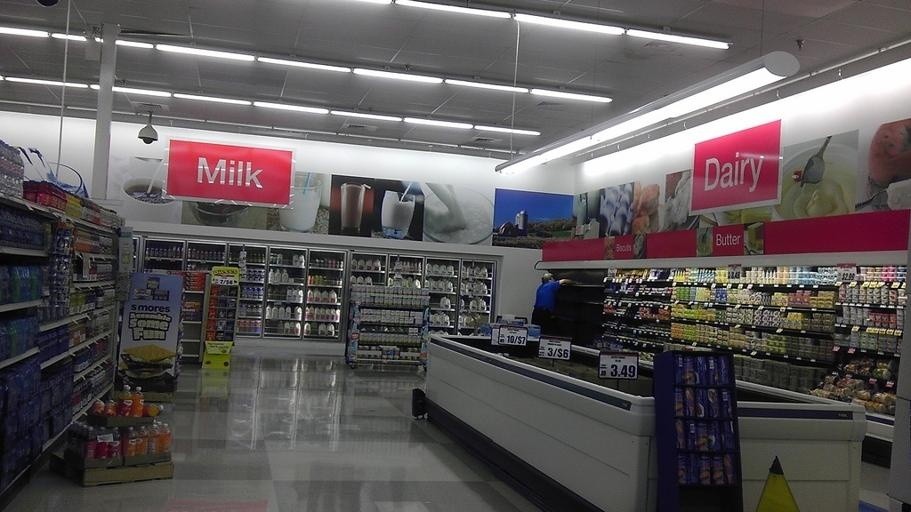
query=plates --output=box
[697,231,713,256]
[634,234,647,258]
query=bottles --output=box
[268,285,304,303]
[277,321,301,337]
[71,338,115,415]
[66,384,171,456]
[308,274,343,286]
[269,268,289,283]
[1,140,26,197]
[265,304,303,320]
[306,305,341,322]
[66,286,115,314]
[72,260,114,282]
[25,179,118,230]
[248,269,266,282]
[305,323,335,337]
[237,304,262,333]
[0,208,50,493]
[69,310,111,345]
[145,247,284,263]
[74,224,115,255]
[291,253,305,267]
[307,288,338,303]
[239,286,264,300]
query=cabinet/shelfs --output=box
[1,197,120,502]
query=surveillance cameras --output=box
[138,124,158,144]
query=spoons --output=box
[800,136,832,186]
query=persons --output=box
[532,273,581,330]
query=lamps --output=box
[492,1,800,177]
[179,288,205,359]
[137,111,159,145]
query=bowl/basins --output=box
[774,143,858,221]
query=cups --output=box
[423,189,495,246]
[381,191,414,240]
[278,175,324,233]
[122,180,181,224]
[339,183,366,235]
[746,222,765,254]
[187,202,249,225]
